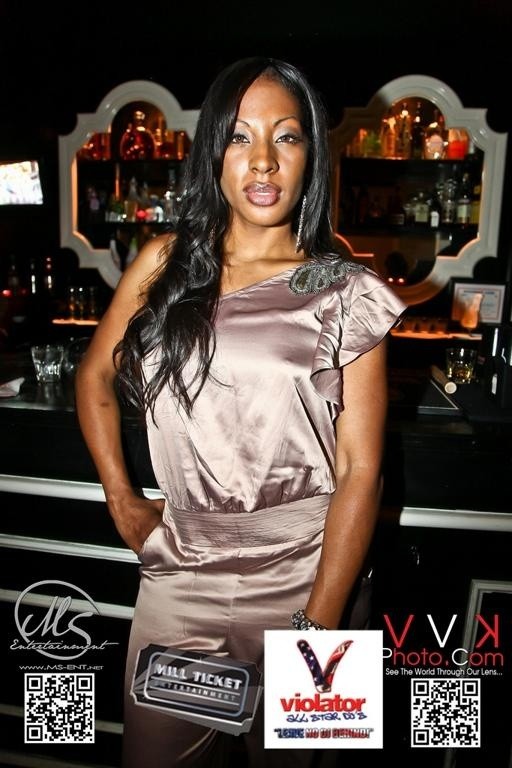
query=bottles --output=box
[341,100,482,229]
[1,254,100,328]
[83,109,191,269]
[483,327,507,404]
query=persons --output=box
[73,54,408,768]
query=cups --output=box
[446,348,477,386]
[32,345,64,384]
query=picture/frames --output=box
[450,281,505,324]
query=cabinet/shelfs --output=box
[73,153,191,229]
[334,151,480,239]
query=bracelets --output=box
[289,608,326,632]
